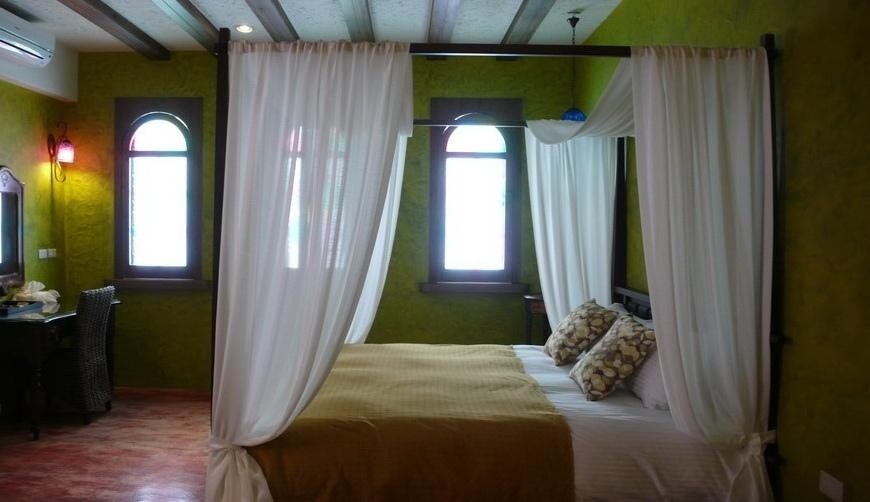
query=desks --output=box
[0,299,123,440]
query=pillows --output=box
[540,297,703,412]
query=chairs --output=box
[9,279,115,425]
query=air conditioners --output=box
[0,7,56,68]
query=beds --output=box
[210,285,775,502]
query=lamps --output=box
[561,18,588,120]
[47,123,77,183]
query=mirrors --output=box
[0,167,24,291]
